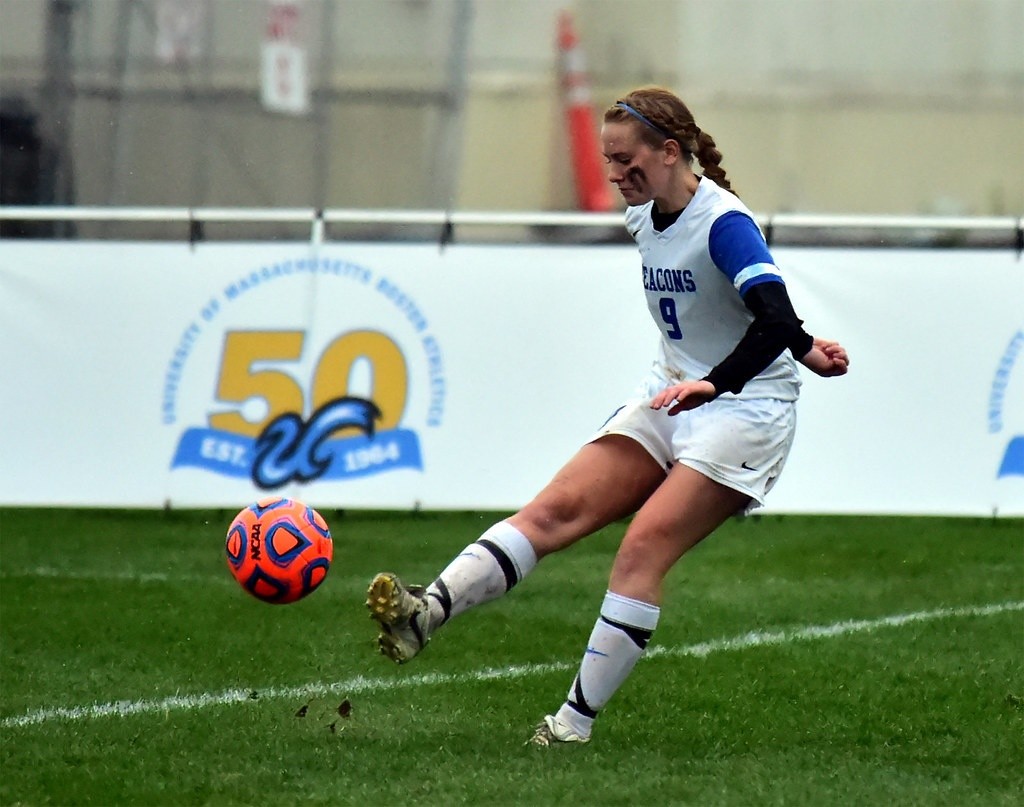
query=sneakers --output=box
[519,713,597,752]
[363,571,432,666]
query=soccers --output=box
[224,496,334,604]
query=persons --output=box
[364,85,850,749]
